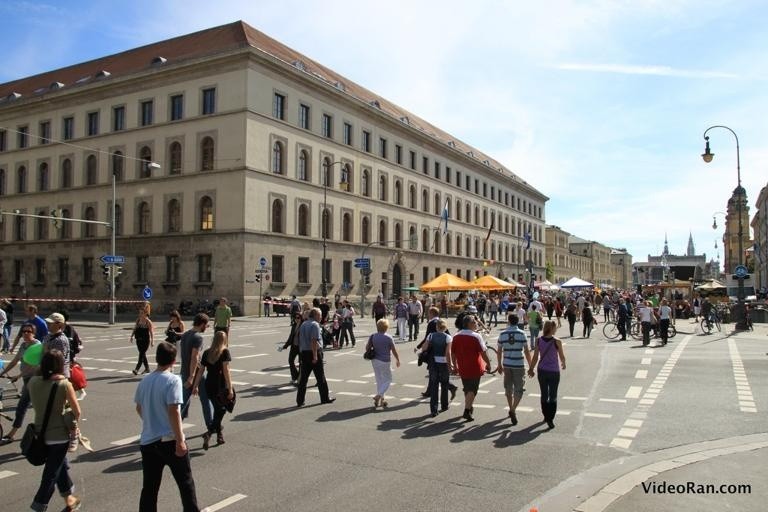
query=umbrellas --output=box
[419,271,526,318]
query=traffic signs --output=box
[104,256,122,262]
[354,258,369,264]
[354,264,369,269]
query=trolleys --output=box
[0,375,16,444]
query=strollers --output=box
[320,319,341,350]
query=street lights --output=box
[712,234,726,277]
[710,210,731,275]
[320,162,349,309]
[701,124,750,332]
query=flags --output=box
[441,200,449,232]
[486,211,494,241]
[525,222,530,249]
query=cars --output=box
[727,273,768,311]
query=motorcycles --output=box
[178,298,220,317]
[96,302,124,312]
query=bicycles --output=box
[449,329,499,376]
[601,309,675,343]
[699,303,754,335]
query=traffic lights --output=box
[117,266,122,276]
[104,265,109,276]
[256,275,259,282]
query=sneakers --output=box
[68,496,83,511]
[289,375,336,407]
[2,436,13,442]
[323,314,669,348]
[132,369,149,375]
[373,383,558,428]
[202,425,225,450]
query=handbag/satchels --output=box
[69,362,87,390]
[363,349,377,360]
[216,387,238,413]
[19,420,51,467]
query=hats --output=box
[45,312,66,325]
